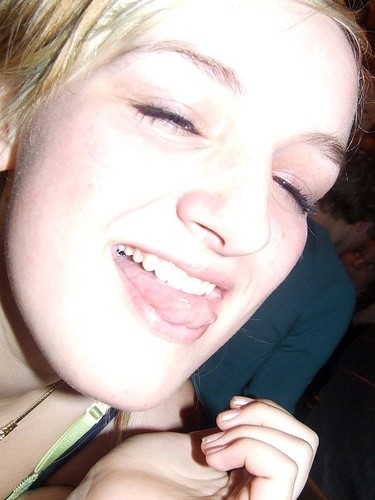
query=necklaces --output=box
[0,380,65,443]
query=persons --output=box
[192,144,375,424]
[0,0,366,500]
